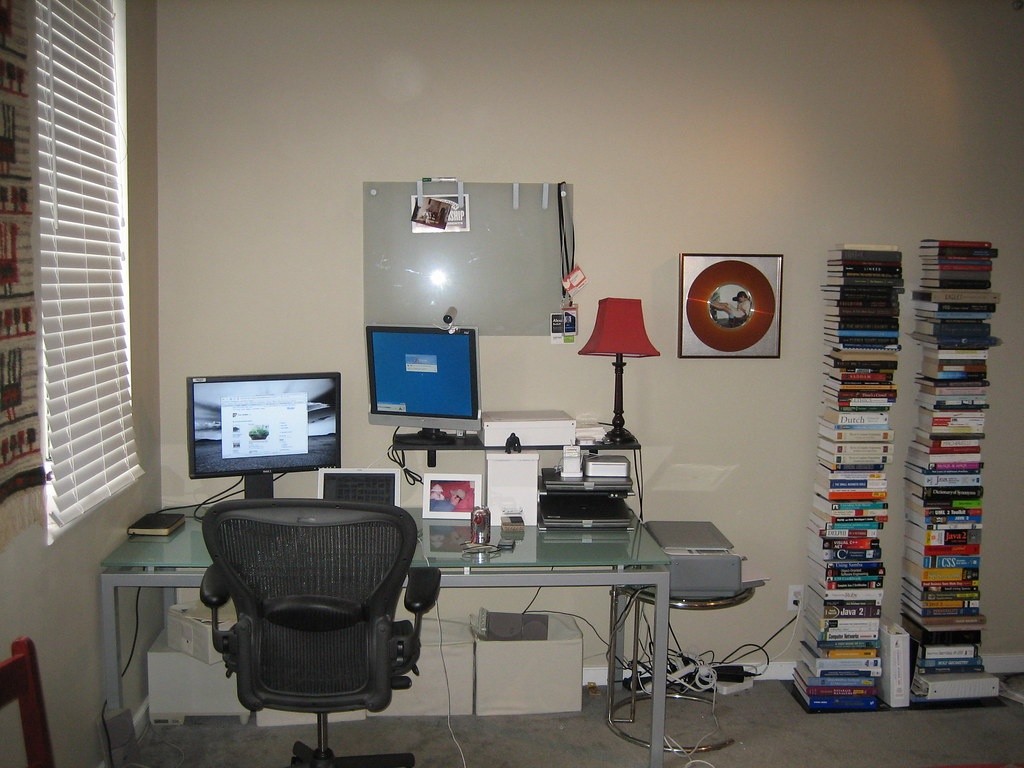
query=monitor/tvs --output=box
[187,372,342,500]
[364,323,482,447]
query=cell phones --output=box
[498,537,515,548]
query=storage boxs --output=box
[167,599,238,665]
[256,706,369,727]
[367,620,475,717]
[477,410,577,447]
[474,616,584,716]
[148,630,251,725]
[485,450,540,525]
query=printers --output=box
[643,520,765,599]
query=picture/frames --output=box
[677,252,784,359]
[318,468,402,507]
[423,473,483,519]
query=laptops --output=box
[318,469,401,508]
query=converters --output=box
[716,666,744,683]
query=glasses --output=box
[460,542,502,553]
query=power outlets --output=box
[787,585,803,610]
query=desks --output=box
[100,505,672,768]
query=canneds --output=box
[471,505,491,544]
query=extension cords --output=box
[716,677,754,695]
[643,662,695,692]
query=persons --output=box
[430,489,465,511]
[430,484,444,500]
[709,291,752,326]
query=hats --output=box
[450,489,465,500]
[732,292,748,301]
[431,484,443,491]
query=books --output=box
[903,240,1002,697]
[793,251,904,712]
[128,514,184,535]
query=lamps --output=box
[577,297,660,445]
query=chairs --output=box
[0,636,56,768]
[199,498,442,768]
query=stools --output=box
[606,565,755,753]
